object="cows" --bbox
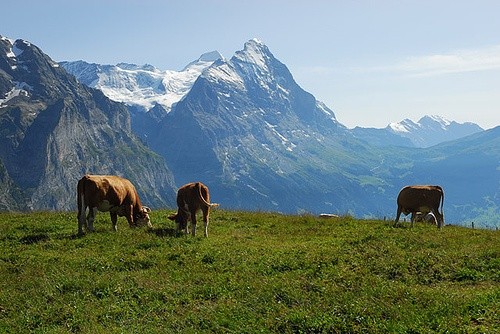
[168,181,220,238]
[392,184,445,230]
[76,173,153,234]
